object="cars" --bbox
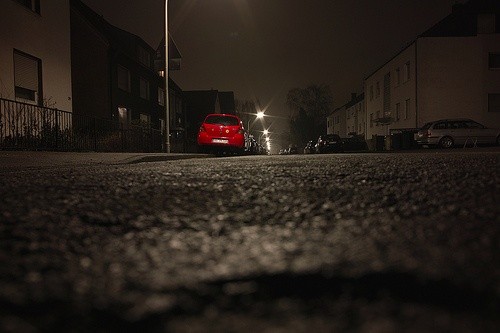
[197,113,266,156]
[278,135,343,154]
[415,118,499,150]
